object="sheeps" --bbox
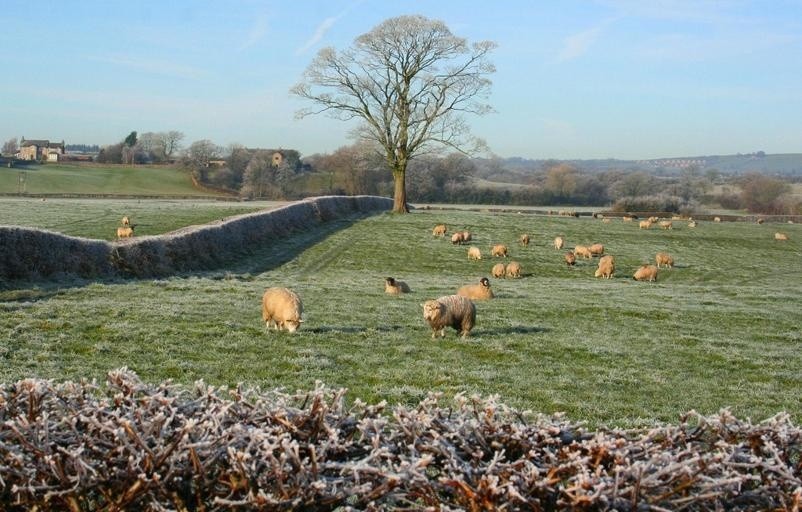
[451,229,614,280]
[432,223,448,237]
[458,277,495,300]
[623,214,698,231]
[116,216,137,240]
[774,231,789,241]
[420,295,476,339]
[385,277,411,294]
[262,286,305,335]
[632,251,675,282]
